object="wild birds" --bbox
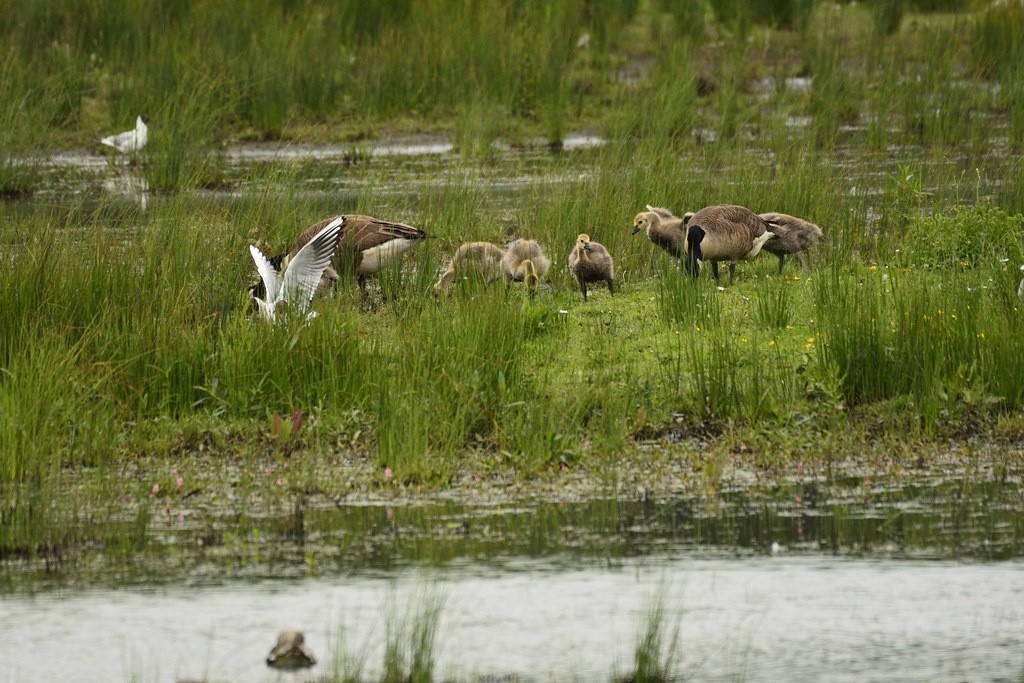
[631,205,823,288]
[433,233,550,299]
[247,214,438,331]
[568,234,616,303]
[101,111,152,155]
